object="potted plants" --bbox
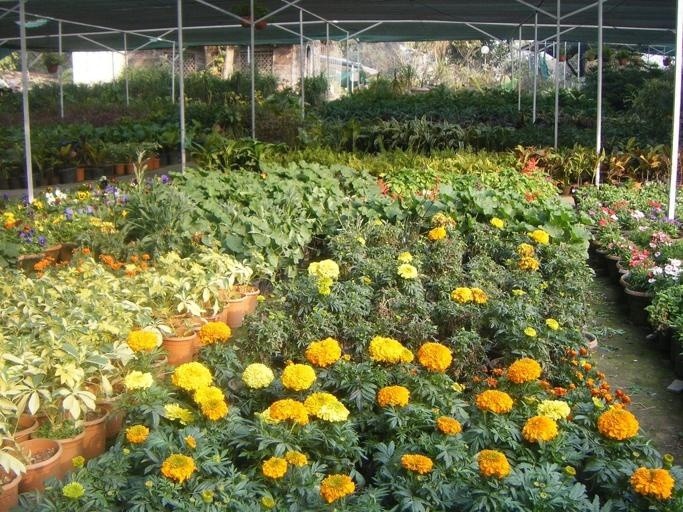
[0,122,197,190]
[559,43,629,66]
[539,136,683,196]
[41,50,64,74]
